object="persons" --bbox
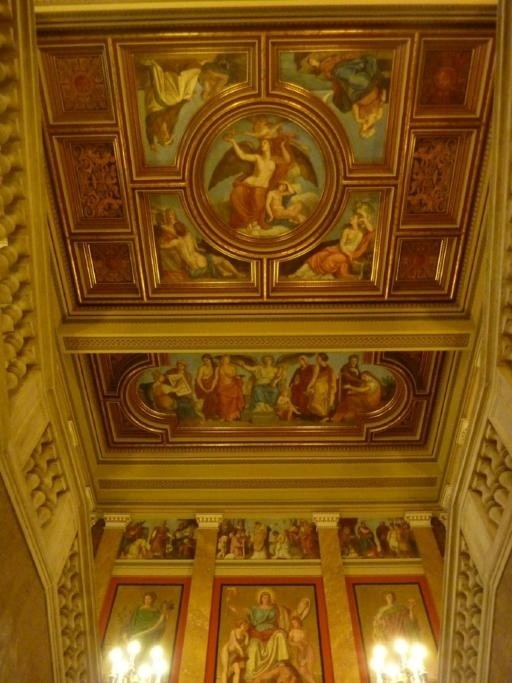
[121,590,166,683]
[337,517,414,559]
[220,592,319,683]
[118,519,200,561]
[149,351,383,425]
[216,519,322,560]
[371,591,428,683]
